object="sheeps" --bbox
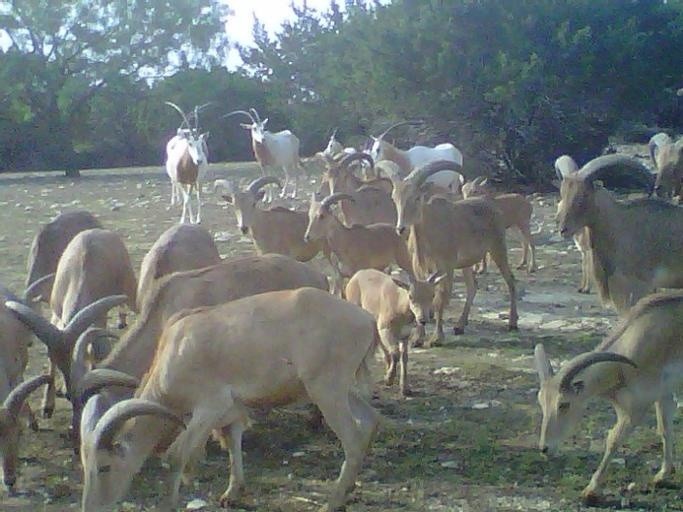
[3,228,138,420]
[555,131,683,312]
[80,287,380,510]
[0,289,52,498]
[161,101,537,291]
[341,267,449,396]
[375,158,520,349]
[67,253,330,472]
[534,291,683,498]
